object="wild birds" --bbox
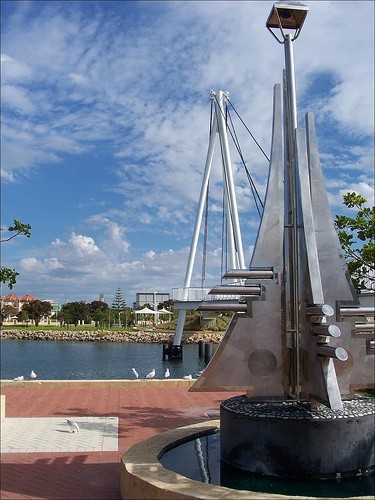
[164,368,170,379]
[131,367,139,379]
[13,376,24,381]
[144,369,156,379]
[30,370,37,381]
[183,374,193,379]
